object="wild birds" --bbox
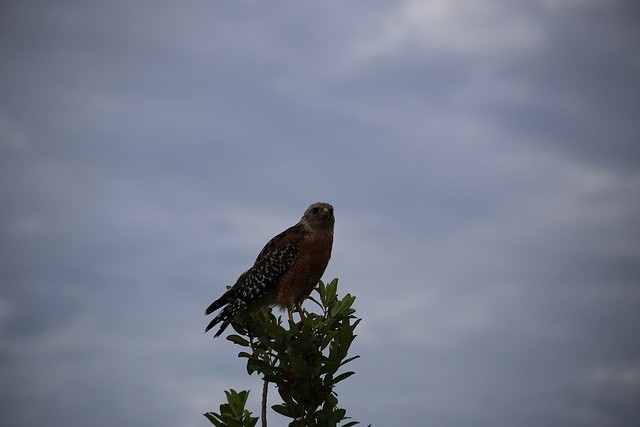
[204,202,339,343]
